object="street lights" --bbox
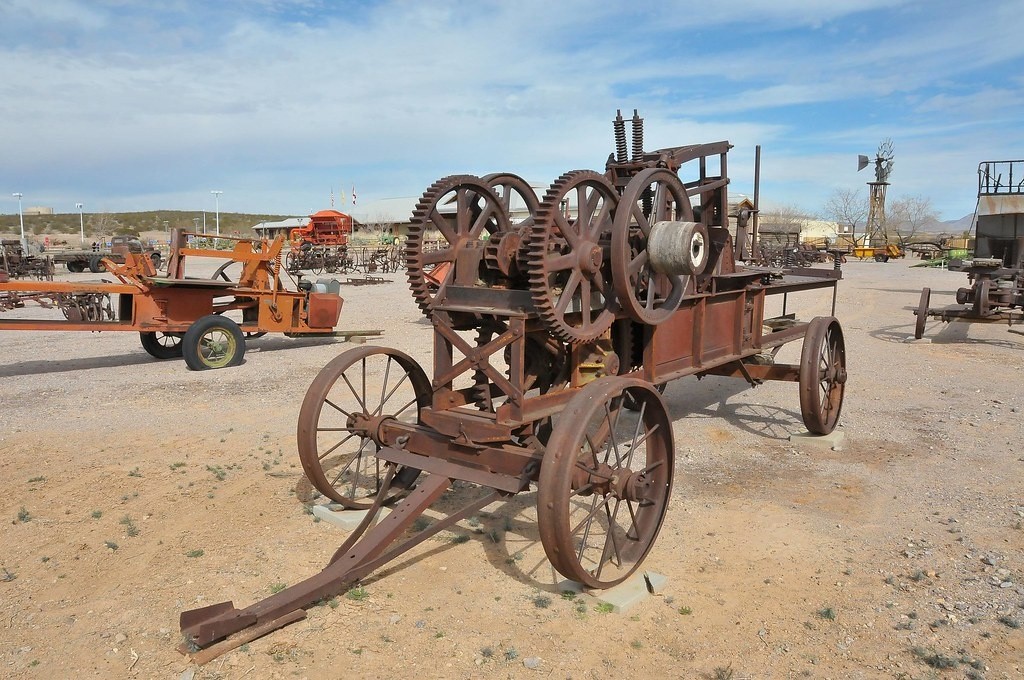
[12,192,27,256]
[210,190,222,237]
[75,201,85,242]
